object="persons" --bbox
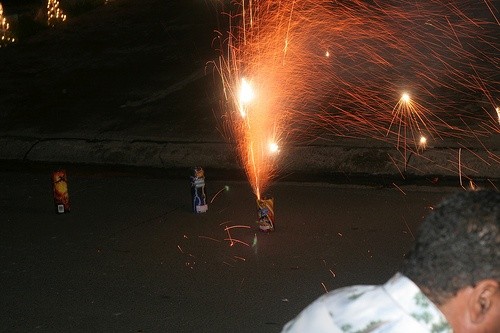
[281,188,500,332]
